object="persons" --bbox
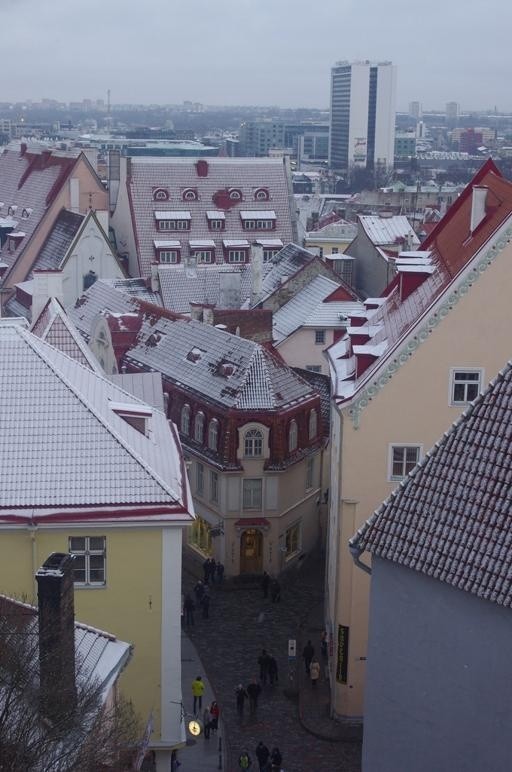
[262,570,270,598]
[233,684,248,719]
[191,676,204,714]
[309,658,320,689]
[271,579,281,603]
[255,741,269,772]
[239,749,253,772]
[204,706,213,739]
[210,701,219,733]
[258,649,270,683]
[183,555,224,626]
[247,679,261,711]
[271,748,282,772]
[303,640,314,673]
[269,658,278,688]
